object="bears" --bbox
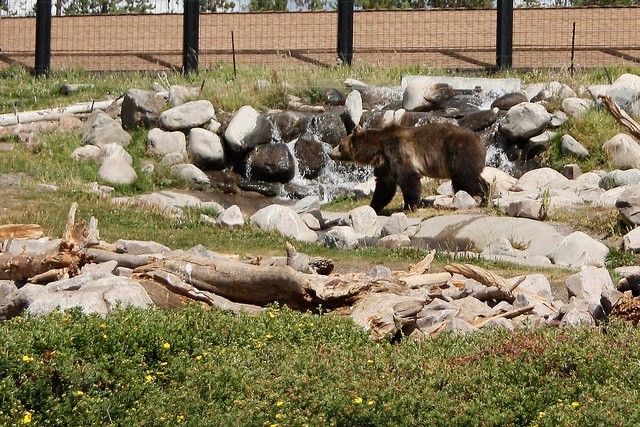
[326,122,491,217]
